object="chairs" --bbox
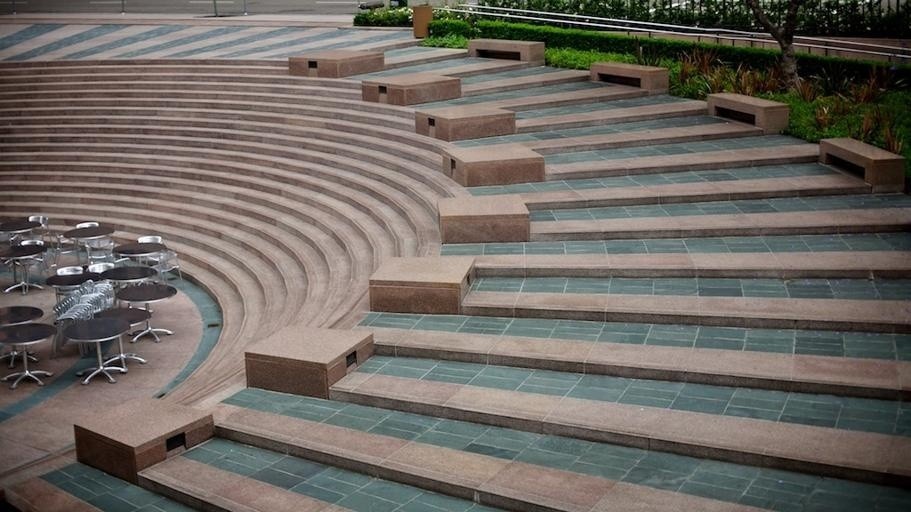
[0,212,185,391]
